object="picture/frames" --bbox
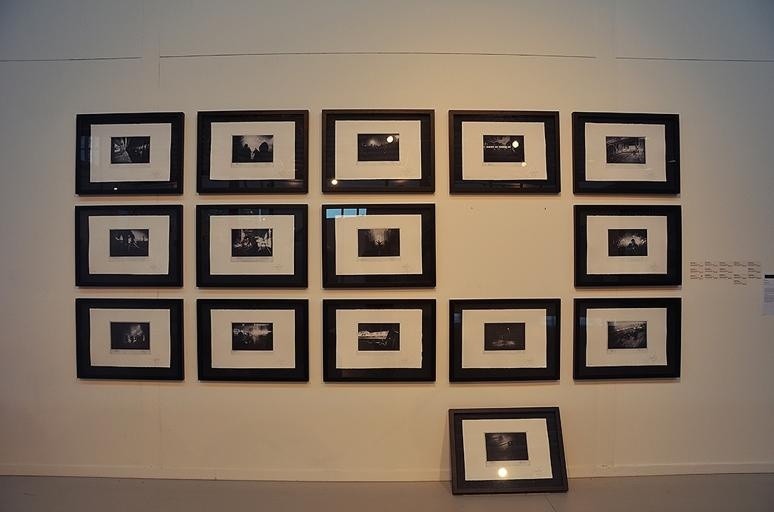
[448,110,561,195]
[320,203,438,286]
[572,109,681,197]
[74,204,183,287]
[74,111,184,195]
[195,204,309,289]
[74,296,183,381]
[573,204,682,287]
[572,296,682,380]
[323,298,435,383]
[448,408,570,496]
[322,109,434,192]
[448,297,560,380]
[196,110,307,194]
[197,297,309,384]
